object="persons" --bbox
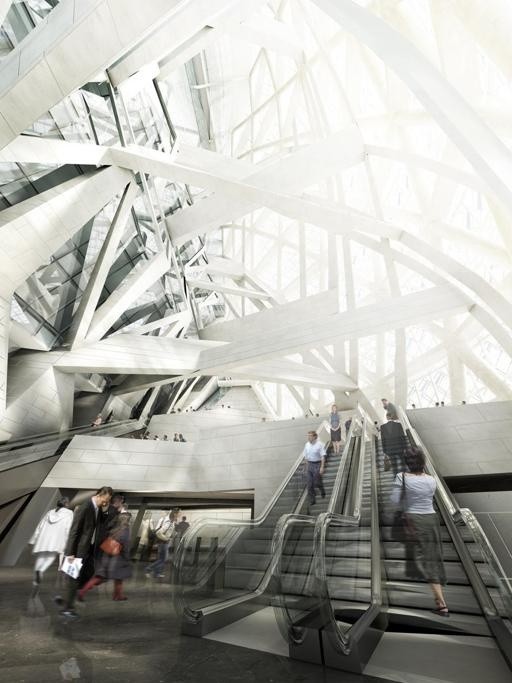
[388,445,451,617]
[381,398,399,424]
[379,412,409,478]
[103,414,114,435]
[303,429,326,506]
[344,414,352,436]
[129,430,185,441]
[90,412,103,436]
[25,484,191,622]
[374,421,381,432]
[169,403,231,414]
[326,403,342,455]
